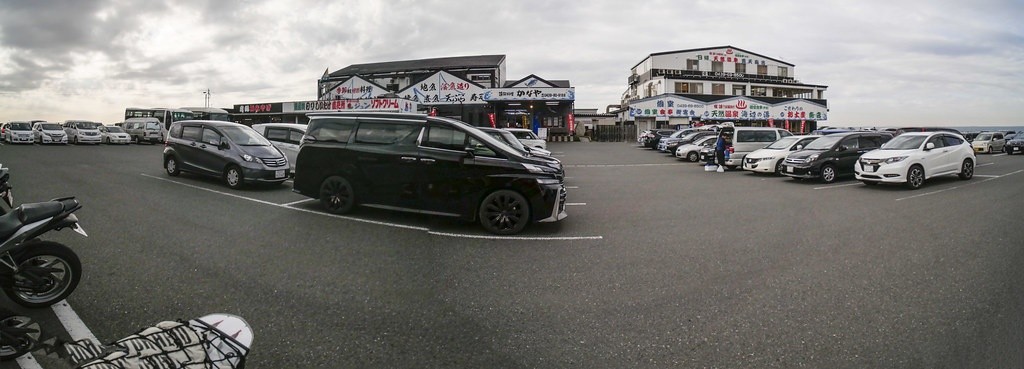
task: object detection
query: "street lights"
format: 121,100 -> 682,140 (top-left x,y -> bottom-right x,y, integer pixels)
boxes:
203,91 -> 207,108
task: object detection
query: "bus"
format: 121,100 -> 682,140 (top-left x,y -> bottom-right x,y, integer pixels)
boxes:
124,107 -> 230,144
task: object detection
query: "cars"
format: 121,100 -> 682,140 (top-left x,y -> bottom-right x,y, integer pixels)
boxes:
0,117 -> 162,145
637,124 -> 1024,190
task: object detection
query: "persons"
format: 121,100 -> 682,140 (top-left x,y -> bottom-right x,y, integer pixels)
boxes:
716,133 -> 729,170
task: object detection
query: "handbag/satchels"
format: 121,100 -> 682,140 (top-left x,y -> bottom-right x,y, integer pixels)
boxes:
717,164 -> 724,172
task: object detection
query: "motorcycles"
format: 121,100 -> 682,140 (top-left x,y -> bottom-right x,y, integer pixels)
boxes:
0,164 -> 88,311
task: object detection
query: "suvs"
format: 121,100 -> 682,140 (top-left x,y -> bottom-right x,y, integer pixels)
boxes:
249,123 -> 336,179
163,120 -> 290,189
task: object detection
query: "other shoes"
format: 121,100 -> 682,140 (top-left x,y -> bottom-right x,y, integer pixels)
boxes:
724,166 -> 728,169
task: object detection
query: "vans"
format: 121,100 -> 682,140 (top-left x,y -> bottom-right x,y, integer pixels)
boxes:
290,113 -> 568,236
